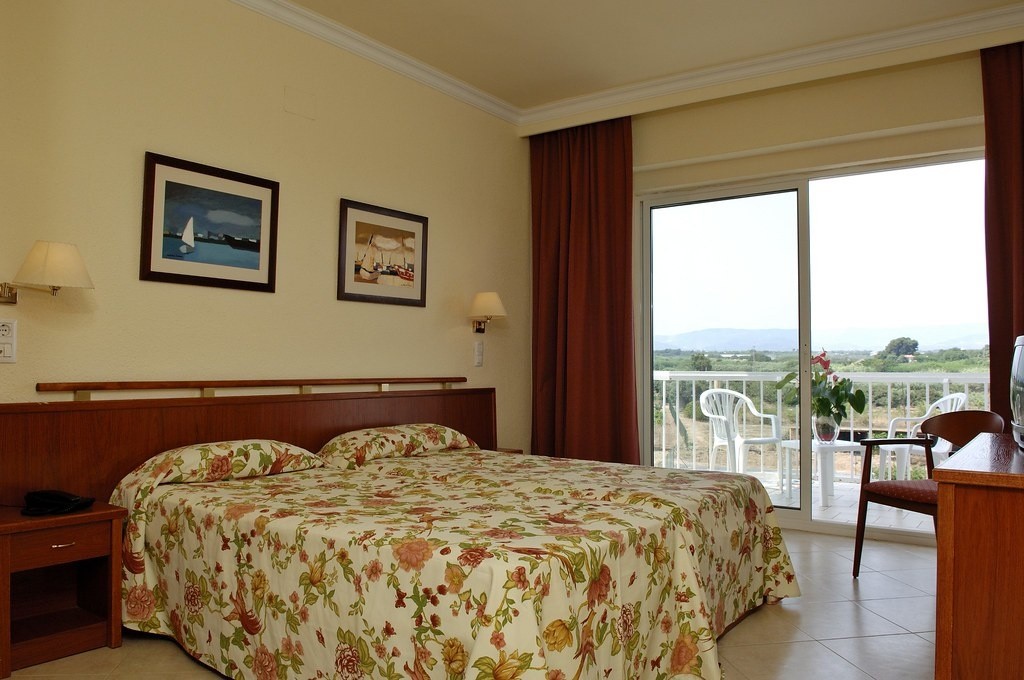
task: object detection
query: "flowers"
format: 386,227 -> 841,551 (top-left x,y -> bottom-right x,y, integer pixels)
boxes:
774,350 -> 867,426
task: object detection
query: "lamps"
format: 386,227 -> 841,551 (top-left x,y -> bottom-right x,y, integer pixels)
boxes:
0,239 -> 95,306
466,291 -> 508,334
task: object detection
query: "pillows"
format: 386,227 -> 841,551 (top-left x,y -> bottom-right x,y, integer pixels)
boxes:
315,423 -> 480,468
130,438 -> 323,484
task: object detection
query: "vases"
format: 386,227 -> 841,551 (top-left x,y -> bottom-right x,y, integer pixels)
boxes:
811,413 -> 840,446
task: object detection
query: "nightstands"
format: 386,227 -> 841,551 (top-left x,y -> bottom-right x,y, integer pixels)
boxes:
1,502 -> 127,679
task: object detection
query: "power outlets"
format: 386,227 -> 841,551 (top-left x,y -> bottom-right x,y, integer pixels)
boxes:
0,318 -> 17,343
474,341 -> 485,356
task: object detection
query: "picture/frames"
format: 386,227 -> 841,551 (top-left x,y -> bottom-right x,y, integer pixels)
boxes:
139,152 -> 280,295
337,197 -> 429,308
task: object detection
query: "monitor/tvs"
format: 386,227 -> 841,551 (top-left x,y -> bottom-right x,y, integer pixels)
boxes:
1009,335 -> 1024,451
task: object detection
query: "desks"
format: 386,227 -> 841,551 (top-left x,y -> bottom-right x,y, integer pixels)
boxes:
778,439 -> 874,508
932,430 -> 1023,680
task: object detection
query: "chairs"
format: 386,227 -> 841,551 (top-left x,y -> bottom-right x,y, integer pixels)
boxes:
877,393 -> 967,484
699,389 -> 785,491
850,409 -> 1005,579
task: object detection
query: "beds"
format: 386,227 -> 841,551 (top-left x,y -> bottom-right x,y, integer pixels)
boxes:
110,422 -> 802,679
